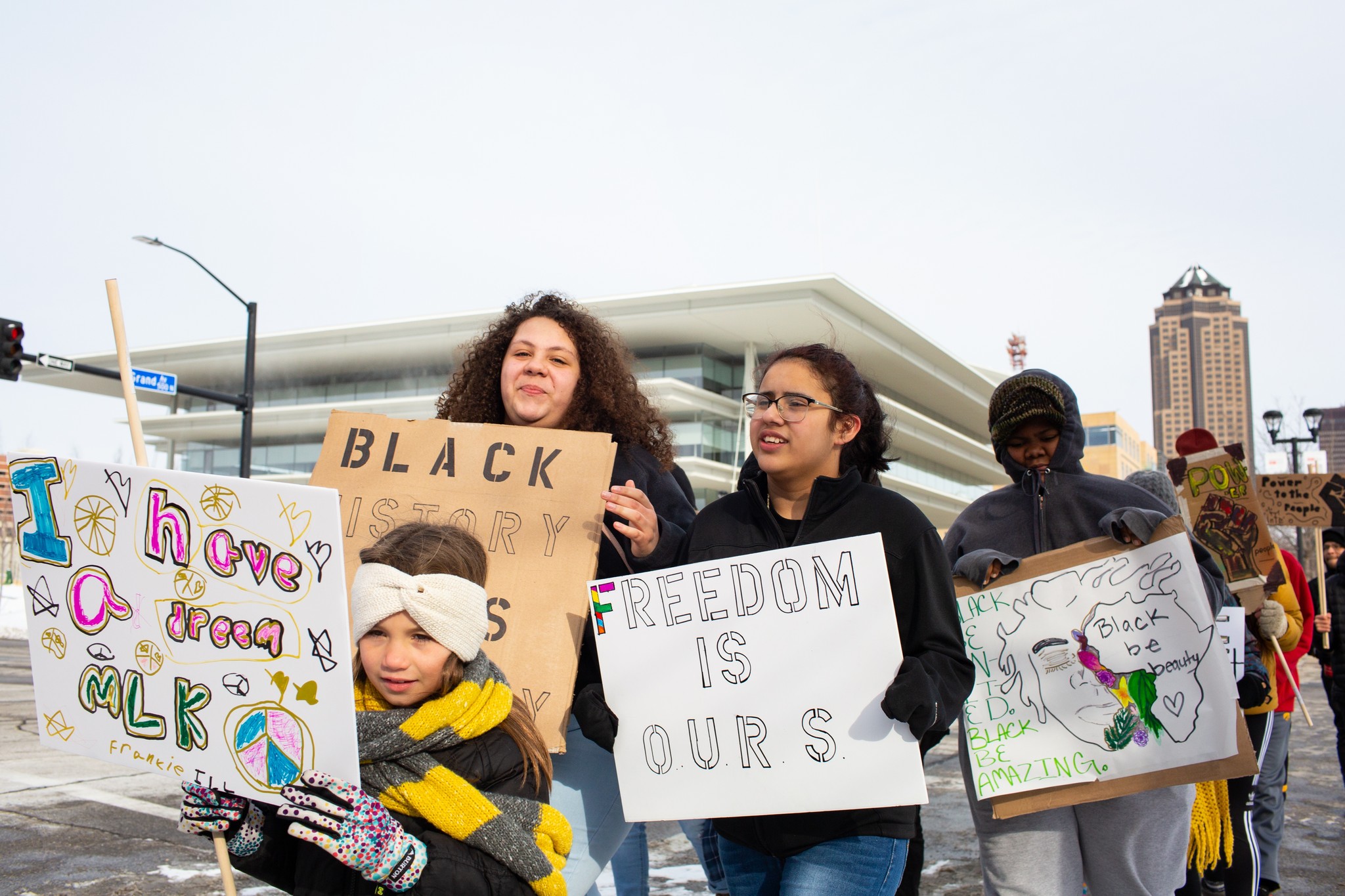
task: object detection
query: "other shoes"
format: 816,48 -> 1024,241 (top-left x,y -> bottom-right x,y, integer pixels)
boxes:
1202,877 -> 1225,892
1257,878 -> 1281,896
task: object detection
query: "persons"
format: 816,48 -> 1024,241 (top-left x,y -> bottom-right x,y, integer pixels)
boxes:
1120,469 -> 1315,896
181,522 -> 572,896
610,820 -> 729,896
673,343 -> 976,896
1307,526 -> 1345,783
434,293 -> 697,896
942,369 -> 1227,896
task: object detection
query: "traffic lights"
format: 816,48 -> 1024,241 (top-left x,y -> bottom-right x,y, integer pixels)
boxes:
0,317 -> 25,382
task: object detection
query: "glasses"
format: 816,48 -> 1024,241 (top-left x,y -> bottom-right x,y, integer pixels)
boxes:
742,393 -> 847,422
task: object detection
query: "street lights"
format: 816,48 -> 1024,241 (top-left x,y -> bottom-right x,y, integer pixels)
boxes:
130,229 -> 259,479
1263,408 -> 1326,572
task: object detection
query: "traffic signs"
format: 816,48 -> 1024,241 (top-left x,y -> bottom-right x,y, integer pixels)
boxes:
36,352 -> 75,374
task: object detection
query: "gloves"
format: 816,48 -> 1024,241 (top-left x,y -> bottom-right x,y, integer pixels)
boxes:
277,771 -> 429,892
1236,672 -> 1268,709
179,780 -> 269,858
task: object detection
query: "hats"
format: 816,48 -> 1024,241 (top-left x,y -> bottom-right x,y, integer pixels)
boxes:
1124,469 -> 1180,515
1322,526 -> 1345,547
991,376 -> 1068,443
